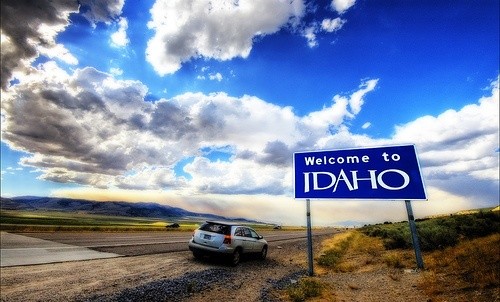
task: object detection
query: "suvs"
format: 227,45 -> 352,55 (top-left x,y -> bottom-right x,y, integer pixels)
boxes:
189,219 -> 269,266
